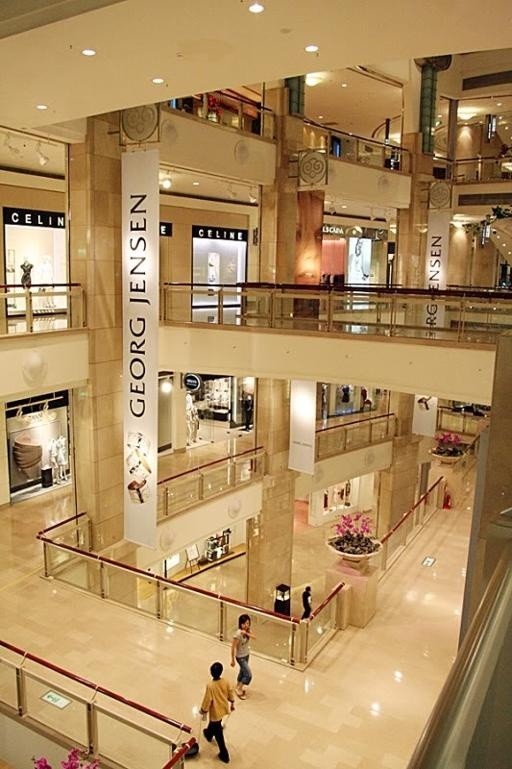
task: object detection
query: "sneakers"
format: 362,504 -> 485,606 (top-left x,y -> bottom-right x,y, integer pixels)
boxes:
235,687 -> 246,700
204,728 -> 212,742
218,753 -> 229,763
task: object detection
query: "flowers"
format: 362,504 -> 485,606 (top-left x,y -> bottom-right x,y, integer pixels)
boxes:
330,509 -> 379,541
432,431 -> 464,456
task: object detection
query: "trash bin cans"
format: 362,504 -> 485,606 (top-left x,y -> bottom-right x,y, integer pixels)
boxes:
42,468 -> 52,488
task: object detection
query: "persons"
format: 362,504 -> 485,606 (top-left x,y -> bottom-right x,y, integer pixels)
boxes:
350,238 -> 364,279
198,661 -> 236,764
239,395 -> 253,430
301,586 -> 313,619
48,439 -> 61,485
57,435 -> 68,481
341,384 -> 349,402
231,613 -> 257,700
20,260 -> 34,290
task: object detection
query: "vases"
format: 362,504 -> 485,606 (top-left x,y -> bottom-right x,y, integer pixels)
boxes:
325,535 -> 383,561
428,448 -> 466,465
